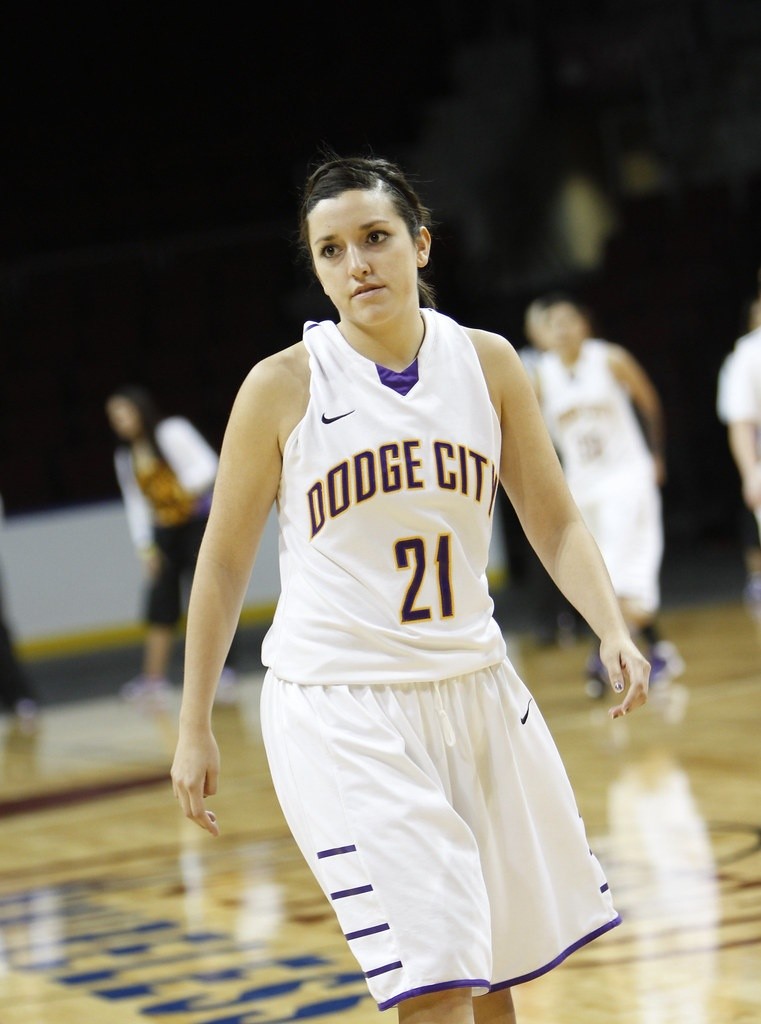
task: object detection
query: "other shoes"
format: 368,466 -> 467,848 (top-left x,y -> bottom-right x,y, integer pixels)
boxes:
587,645 -> 610,701
646,641 -> 681,690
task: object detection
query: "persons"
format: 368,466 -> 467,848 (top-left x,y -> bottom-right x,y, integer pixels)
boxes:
169,159 -> 652,1024
107,385 -> 240,704
718,323 -> 760,518
516,296 -> 684,700
